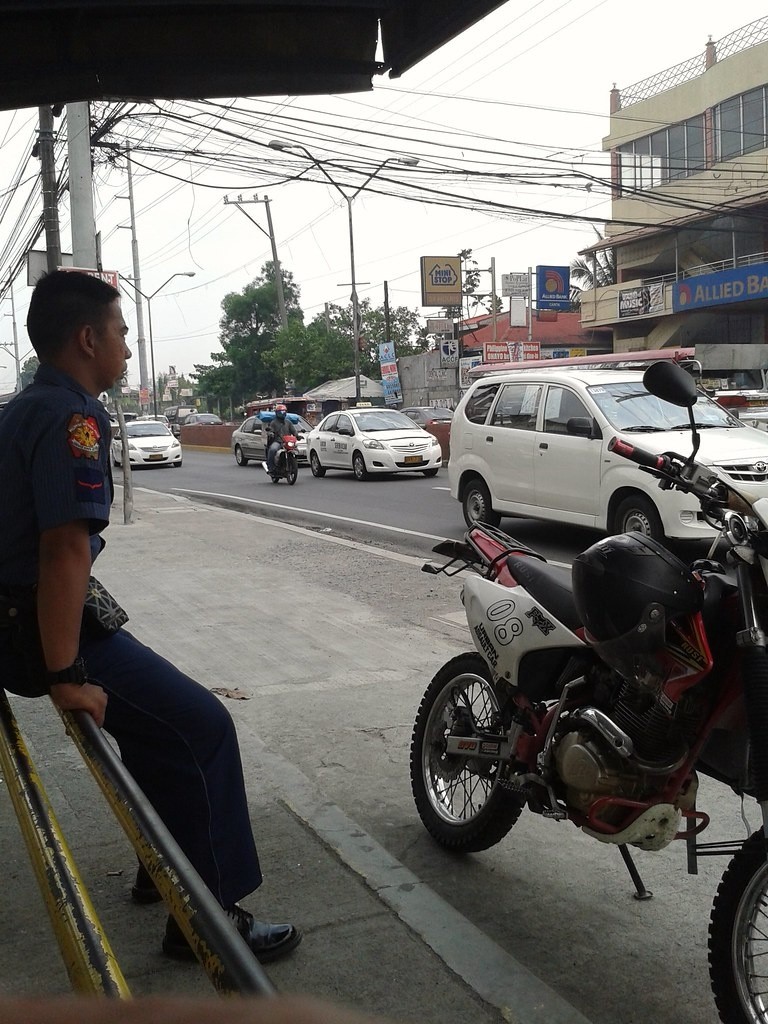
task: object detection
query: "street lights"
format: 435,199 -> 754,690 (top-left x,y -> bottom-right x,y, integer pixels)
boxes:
268,138 -> 421,409
112,271 -> 195,419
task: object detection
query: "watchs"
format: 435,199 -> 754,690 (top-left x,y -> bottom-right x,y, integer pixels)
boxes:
45,657 -> 87,686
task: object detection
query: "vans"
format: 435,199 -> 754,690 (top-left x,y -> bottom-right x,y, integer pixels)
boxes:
446,369 -> 768,548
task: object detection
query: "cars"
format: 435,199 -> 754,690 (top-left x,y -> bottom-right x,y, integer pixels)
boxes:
112,420 -> 183,467
232,412 -> 318,466
108,405 -> 232,429
306,401 -> 444,481
398,406 -> 456,431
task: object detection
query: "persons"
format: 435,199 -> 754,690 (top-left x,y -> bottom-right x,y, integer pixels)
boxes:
0,269 -> 301,962
267,405 -> 305,475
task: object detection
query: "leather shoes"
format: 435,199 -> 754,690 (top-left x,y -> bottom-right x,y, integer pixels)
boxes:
163,903 -> 302,962
131,864 -> 165,904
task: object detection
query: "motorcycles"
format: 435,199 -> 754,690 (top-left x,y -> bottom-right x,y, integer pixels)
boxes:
409,355 -> 768,1024
260,426 -> 306,486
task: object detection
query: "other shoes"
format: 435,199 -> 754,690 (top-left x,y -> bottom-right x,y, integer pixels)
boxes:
268,469 -> 275,473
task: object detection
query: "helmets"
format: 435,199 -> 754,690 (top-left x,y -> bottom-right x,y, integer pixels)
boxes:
276,405 -> 287,418
573,531 -> 704,691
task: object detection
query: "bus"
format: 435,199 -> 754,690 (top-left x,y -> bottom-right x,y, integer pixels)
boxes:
245,397 -> 317,425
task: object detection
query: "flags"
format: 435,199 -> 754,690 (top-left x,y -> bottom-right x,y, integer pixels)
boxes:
379,342 -> 404,406
